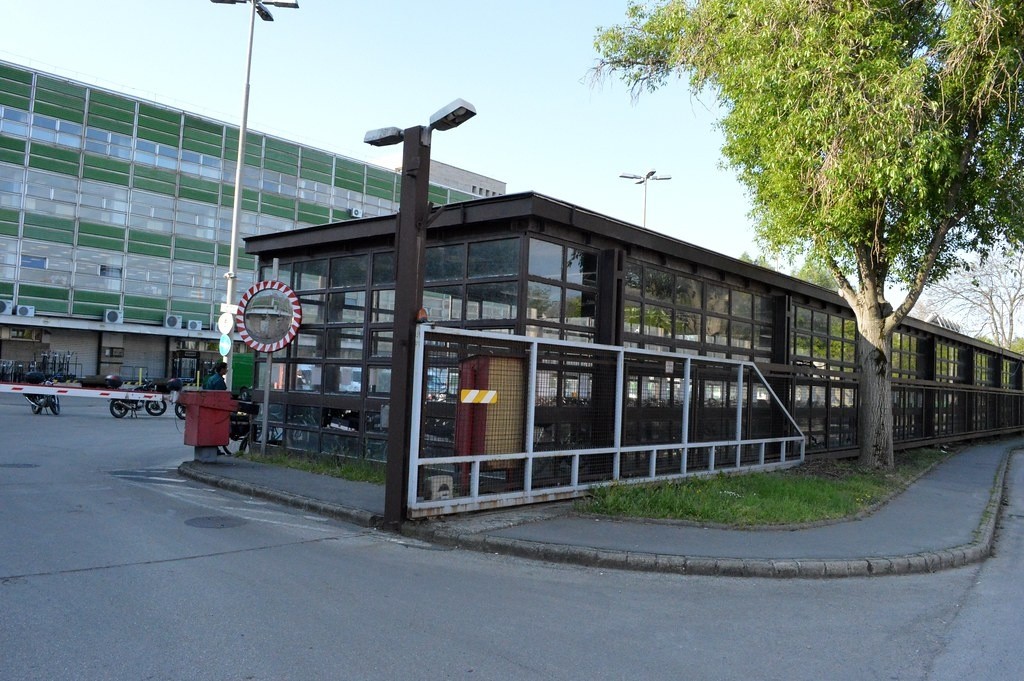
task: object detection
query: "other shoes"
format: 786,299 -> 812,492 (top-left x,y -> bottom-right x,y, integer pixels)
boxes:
217,450 -> 226,455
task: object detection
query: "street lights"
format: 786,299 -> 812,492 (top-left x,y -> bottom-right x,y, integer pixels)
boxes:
210,0 -> 299,387
363,98 -> 477,531
619,168 -> 672,228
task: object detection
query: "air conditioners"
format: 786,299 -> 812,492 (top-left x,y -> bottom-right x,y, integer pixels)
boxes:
163,315 -> 183,329
213,322 -> 220,332
103,308 -> 123,324
187,320 -> 203,330
15,305 -> 34,316
0,299 -> 12,315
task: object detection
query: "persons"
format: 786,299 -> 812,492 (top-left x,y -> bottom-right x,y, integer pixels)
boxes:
203,361 -> 233,456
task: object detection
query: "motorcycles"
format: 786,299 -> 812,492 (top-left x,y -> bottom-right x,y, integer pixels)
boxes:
104,370 -> 167,419
23,371 -> 64,415
